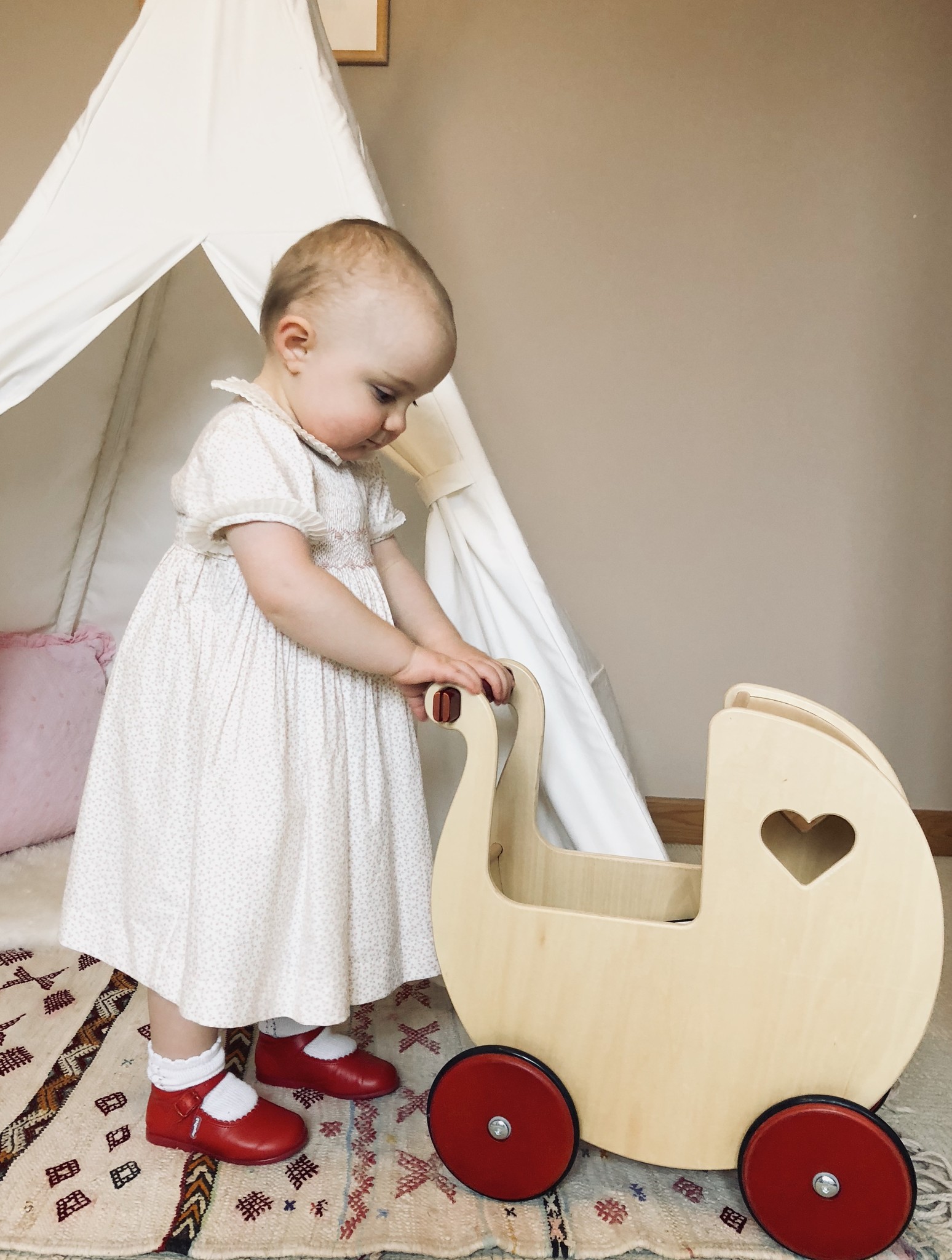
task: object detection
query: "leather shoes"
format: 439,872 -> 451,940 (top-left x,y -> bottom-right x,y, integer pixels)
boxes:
145,1070 -> 310,1167
255,1027 -> 400,1099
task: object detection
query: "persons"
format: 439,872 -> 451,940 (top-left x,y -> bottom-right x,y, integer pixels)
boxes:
50,214 -> 516,1162
